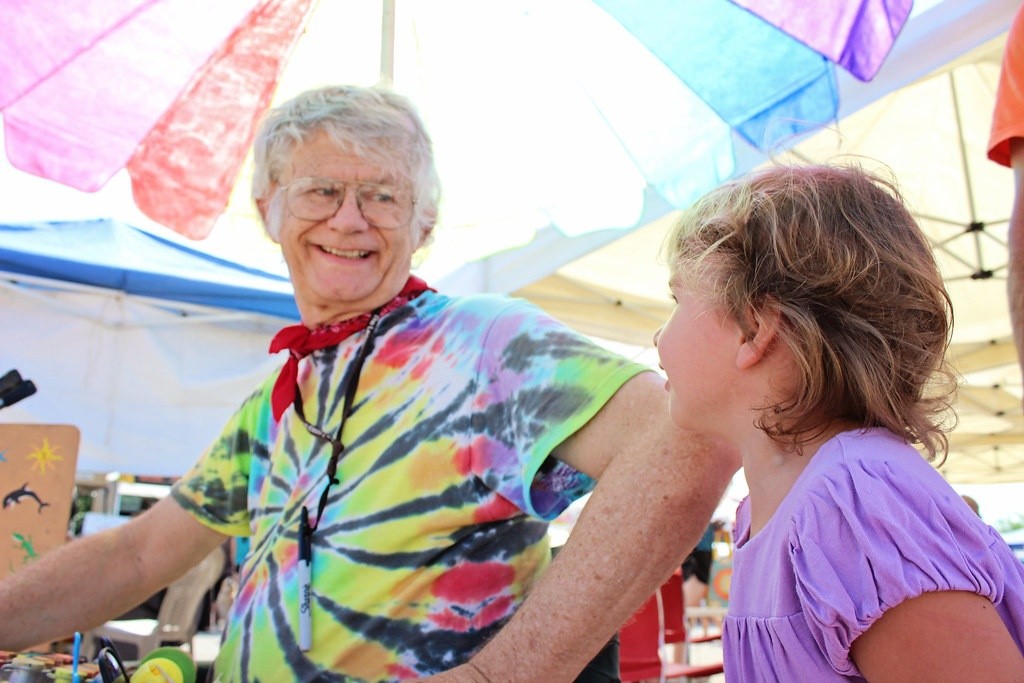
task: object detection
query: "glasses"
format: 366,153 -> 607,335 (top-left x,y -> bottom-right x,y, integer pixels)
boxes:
279,177 -> 419,230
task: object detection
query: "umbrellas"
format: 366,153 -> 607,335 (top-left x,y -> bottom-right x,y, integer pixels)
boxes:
1,0 -> 911,286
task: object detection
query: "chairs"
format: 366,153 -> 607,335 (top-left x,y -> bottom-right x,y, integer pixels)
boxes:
618,563 -> 725,683
82,546 -> 226,672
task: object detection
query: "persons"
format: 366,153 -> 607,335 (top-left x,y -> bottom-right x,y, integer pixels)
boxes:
654,164 -> 1023,683
0,83 -> 742,681
986,10 -> 1023,390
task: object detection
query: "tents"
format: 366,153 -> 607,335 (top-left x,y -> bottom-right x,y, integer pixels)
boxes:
1,215 -> 305,483
